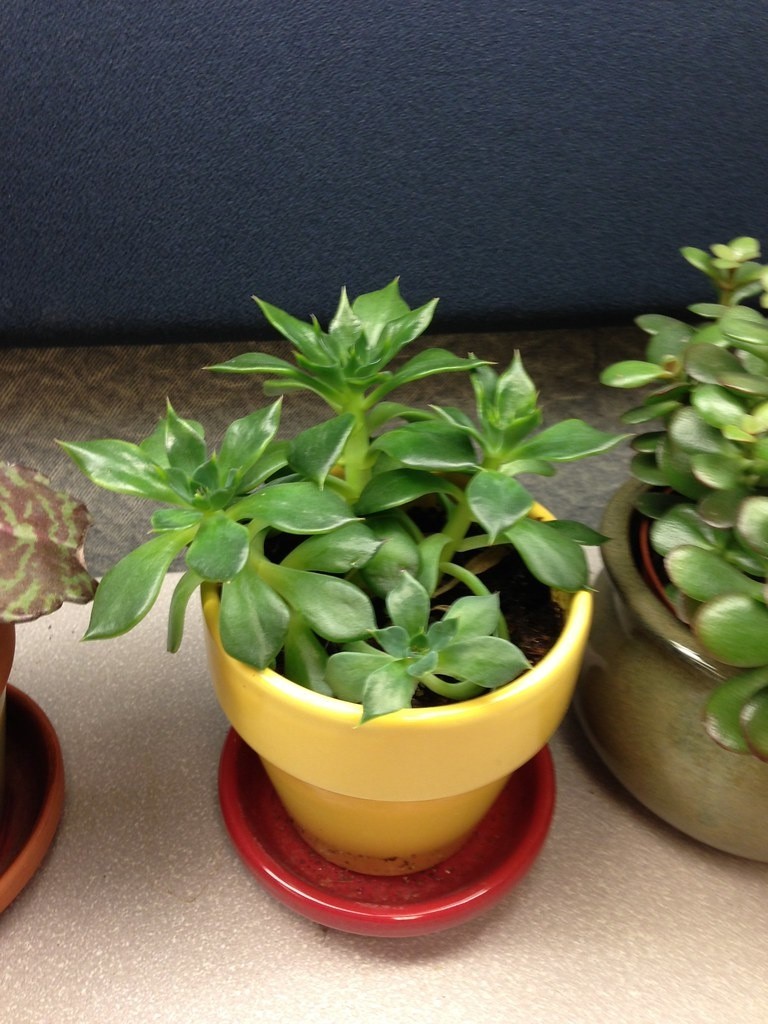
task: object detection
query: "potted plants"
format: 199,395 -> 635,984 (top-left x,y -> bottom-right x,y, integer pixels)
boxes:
572,233 -> 768,864
46,274 -> 637,882
0,461 -> 102,916
